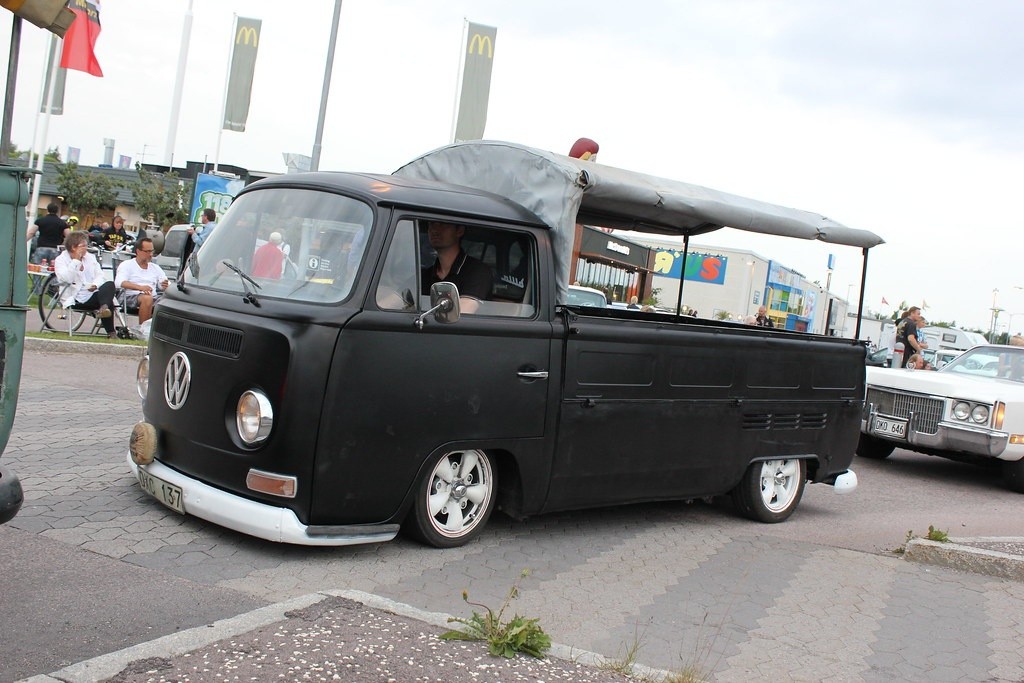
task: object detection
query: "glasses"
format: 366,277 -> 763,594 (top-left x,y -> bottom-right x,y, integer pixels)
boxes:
141,249 -> 155,254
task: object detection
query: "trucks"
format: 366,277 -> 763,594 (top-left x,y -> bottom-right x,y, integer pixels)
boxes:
128,140 -> 884,548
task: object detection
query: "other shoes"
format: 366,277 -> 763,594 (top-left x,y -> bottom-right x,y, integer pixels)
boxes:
96,309 -> 121,340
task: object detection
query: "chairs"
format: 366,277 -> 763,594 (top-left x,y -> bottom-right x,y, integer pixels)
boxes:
40,282 -> 110,337
481,272 -> 528,316
116,287 -> 140,335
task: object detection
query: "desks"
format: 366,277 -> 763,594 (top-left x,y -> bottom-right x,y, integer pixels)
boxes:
28,270 -> 57,302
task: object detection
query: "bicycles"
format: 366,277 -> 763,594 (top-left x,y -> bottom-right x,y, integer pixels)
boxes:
39,239 -> 135,333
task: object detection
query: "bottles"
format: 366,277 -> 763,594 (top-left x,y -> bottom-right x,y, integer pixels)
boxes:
40,258 -> 47,273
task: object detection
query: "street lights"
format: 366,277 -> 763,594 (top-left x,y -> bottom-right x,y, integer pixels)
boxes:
989,307 -> 1004,343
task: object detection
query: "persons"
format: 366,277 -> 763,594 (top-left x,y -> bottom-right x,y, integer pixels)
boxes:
915,316 -> 928,358
66,215 -> 79,233
376,221 -> 483,313
923,361 -> 931,369
27,203 -> 70,294
274,228 -> 290,279
887,318 -> 903,368
102,222 -> 109,237
639,305 -> 656,313
895,306 -> 928,368
692,310 -> 697,317
250,231 -> 282,280
688,309 -> 693,315
627,296 -> 640,309
602,288 -> 612,304
755,305 -> 774,327
55,232 -> 120,340
744,315 -> 758,326
115,238 -> 171,324
901,312 -> 908,319
88,220 -> 103,233
907,353 -> 923,369
186,209 -> 216,254
104,215 -> 126,279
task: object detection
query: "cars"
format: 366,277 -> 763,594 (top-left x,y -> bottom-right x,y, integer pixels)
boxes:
858,343 -> 1022,488
156,222 -> 192,284
